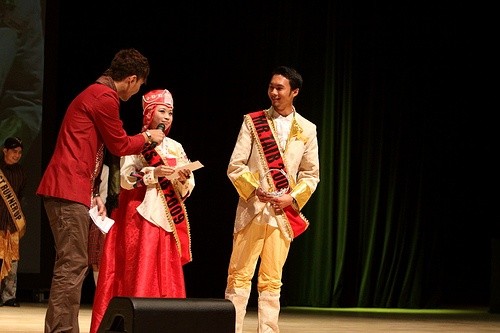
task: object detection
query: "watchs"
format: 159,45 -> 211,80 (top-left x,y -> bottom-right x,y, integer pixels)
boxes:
145,130 -> 151,143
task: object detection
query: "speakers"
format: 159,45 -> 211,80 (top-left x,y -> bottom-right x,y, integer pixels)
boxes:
97,295 -> 236,333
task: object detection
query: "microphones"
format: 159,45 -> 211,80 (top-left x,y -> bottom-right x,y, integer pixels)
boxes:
148,123 -> 165,150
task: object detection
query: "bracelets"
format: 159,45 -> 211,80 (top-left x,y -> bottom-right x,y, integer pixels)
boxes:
93,192 -> 100,196
292,196 -> 296,204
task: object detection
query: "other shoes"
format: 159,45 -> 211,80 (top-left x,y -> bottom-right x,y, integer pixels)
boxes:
4,298 -> 19,307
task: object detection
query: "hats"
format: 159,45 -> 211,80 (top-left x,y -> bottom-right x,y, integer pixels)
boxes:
3,137 -> 23,150
142,89 -> 174,116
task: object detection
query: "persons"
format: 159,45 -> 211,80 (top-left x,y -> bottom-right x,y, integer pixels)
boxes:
82,162 -> 110,289
35,47 -> 152,333
90,87 -> 197,333
0,136 -> 30,307
226,64 -> 320,332
